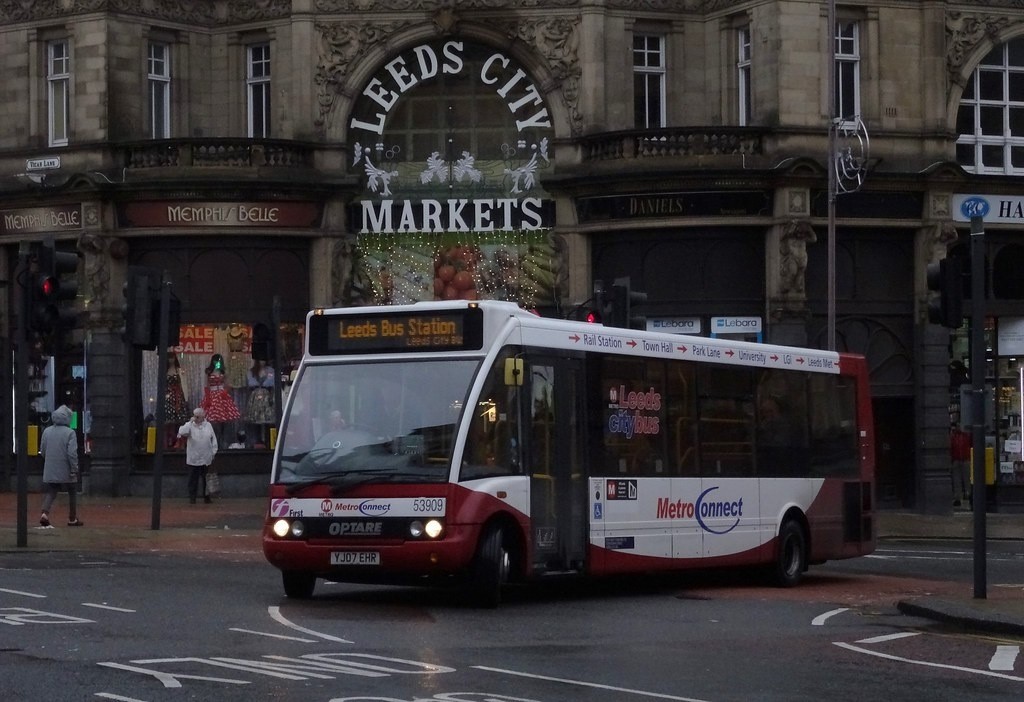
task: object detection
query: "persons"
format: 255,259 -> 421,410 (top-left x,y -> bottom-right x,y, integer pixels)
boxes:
950,424 -> 972,506
167,353 -> 188,423
178,407 -> 218,504
490,420 -> 512,468
464,417 -> 490,466
246,353 -> 275,443
927,223 -> 958,274
227,326 -> 250,405
40,405 -> 85,526
780,218 -> 817,294
201,354 -> 239,447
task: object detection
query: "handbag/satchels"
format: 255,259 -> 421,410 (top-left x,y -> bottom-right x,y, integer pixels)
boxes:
205,473 -> 221,494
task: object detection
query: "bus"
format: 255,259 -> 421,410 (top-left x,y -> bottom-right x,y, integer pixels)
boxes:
262,299 -> 876,610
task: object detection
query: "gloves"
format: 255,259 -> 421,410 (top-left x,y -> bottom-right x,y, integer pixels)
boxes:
70,472 -> 77,477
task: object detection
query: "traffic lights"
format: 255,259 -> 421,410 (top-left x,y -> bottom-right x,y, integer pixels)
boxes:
614,278 -> 648,331
25,259 -> 61,333
573,305 -> 600,323
118,268 -> 163,350
925,257 -> 958,329
268,425 -> 277,451
146,426 -> 156,454
26,425 -> 40,457
48,248 -> 84,331
250,323 -> 274,361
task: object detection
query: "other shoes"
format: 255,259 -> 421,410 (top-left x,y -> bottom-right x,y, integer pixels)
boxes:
68,518 -> 83,526
190,497 -> 195,503
204,497 -> 213,503
40,513 -> 50,526
